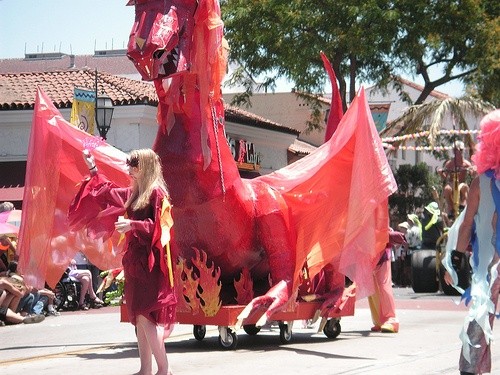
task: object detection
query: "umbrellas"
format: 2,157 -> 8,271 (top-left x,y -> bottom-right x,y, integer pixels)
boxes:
379,97 -> 496,189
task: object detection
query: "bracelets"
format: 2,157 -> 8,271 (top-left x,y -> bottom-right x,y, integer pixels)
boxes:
89,165 -> 96,170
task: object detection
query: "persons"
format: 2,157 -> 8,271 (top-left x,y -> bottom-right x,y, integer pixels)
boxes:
67,148 -> 178,375
0,110 -> 500,375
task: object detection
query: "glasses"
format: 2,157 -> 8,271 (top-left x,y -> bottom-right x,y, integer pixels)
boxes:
126,157 -> 141,167
453,148 -> 464,151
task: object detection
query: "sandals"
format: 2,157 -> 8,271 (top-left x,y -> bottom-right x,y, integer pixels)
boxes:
79,303 -> 89,311
89,297 -> 105,305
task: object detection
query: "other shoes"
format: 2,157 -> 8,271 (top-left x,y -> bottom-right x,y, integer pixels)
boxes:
22,315 -> 45,324
48,310 -> 61,316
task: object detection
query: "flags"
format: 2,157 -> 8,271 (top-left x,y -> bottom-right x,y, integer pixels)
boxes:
70,87 -> 96,136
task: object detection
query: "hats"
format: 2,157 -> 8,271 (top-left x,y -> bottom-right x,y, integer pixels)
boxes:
425,201 -> 440,216
408,213 -> 419,224
398,222 -> 409,229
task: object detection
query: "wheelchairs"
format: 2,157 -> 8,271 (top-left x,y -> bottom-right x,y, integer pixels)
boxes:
45,270 -> 103,312
